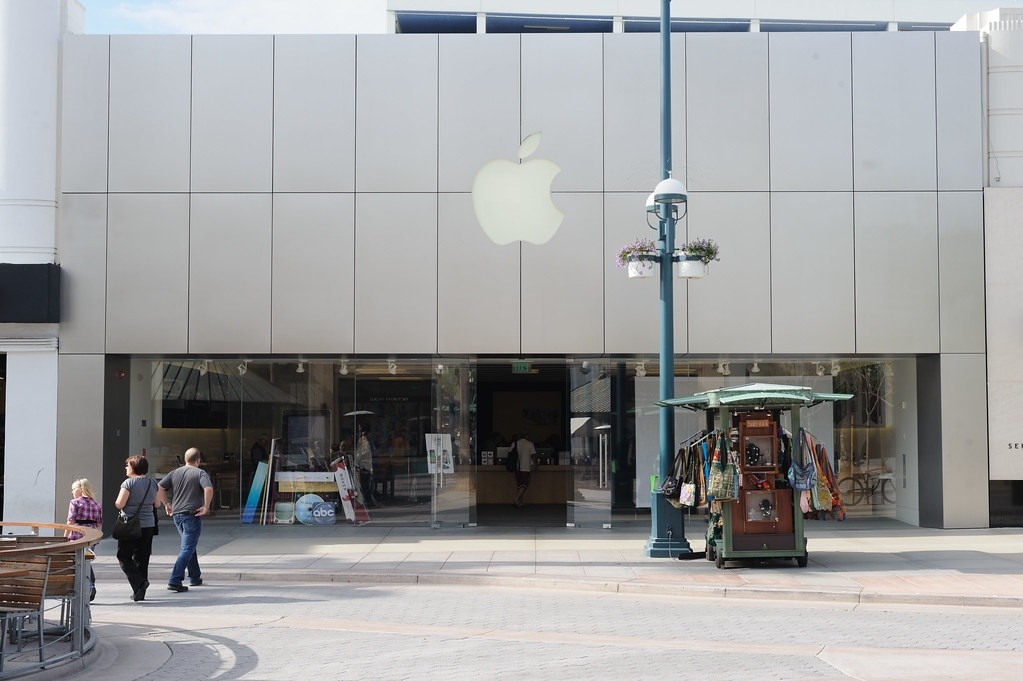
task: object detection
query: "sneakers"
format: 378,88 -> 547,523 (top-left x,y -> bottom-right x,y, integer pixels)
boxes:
190,578 -> 202,586
167,584 -> 188,592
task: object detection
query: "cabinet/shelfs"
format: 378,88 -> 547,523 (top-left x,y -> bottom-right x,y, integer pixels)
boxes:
704,405 -> 806,559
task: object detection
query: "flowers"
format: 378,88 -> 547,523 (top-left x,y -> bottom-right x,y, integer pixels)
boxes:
616,236 -> 659,275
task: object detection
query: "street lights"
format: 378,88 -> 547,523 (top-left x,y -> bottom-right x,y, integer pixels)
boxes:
616,0 -> 722,559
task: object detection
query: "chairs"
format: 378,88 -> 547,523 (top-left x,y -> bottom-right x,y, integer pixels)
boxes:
160,464 -> 174,474
0,555 -> 52,673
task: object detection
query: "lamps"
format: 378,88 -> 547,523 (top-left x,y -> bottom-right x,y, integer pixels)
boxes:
811,361 -> 826,377
435,364 -> 444,375
830,359 -> 841,377
751,363 -> 760,372
386,360 -> 398,375
236,360 -> 254,375
198,360 -> 215,376
722,363 -> 731,376
717,363 -> 725,373
339,360 -> 350,375
634,362 -> 647,377
295,360 -> 310,373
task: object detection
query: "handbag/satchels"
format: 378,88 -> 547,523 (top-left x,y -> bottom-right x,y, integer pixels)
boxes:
705,502 -> 723,546
783,427 -> 847,522
506,440 -> 519,473
112,514 -> 141,540
660,432 -> 741,510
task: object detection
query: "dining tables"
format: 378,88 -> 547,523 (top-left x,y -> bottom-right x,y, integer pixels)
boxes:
198,461 -> 231,511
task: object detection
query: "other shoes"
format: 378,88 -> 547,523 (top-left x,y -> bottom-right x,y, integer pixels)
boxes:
89,587 -> 96,601
513,498 -> 523,506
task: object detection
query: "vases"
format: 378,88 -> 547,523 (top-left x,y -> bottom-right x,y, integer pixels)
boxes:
682,237 -> 720,276
678,259 -> 705,278
627,260 -> 656,279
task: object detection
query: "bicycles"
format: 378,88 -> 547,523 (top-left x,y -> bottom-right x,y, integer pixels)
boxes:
837,458 -> 898,506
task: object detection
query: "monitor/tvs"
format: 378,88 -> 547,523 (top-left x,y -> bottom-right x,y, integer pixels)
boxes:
283,409 -> 331,470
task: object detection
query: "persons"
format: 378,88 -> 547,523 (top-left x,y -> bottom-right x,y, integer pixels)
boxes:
64,477 -> 103,602
158,447 -> 213,592
115,455 -> 161,601
250,429 -> 416,507
509,430 -> 537,507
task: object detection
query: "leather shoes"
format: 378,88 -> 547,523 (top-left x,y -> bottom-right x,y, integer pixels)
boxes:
130,593 -> 144,599
133,581 -> 149,602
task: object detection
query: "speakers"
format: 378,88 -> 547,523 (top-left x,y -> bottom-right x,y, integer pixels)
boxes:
0,263 -> 61,324
